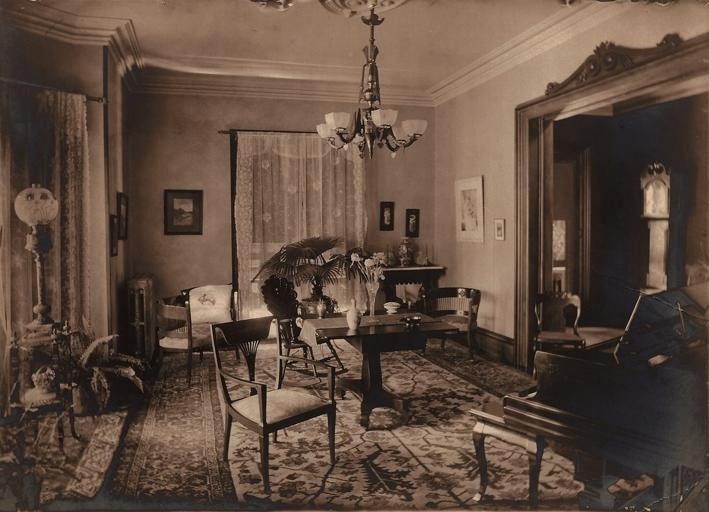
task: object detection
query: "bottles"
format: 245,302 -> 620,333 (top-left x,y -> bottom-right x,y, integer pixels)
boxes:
346,297 -> 361,331
397,237 -> 414,267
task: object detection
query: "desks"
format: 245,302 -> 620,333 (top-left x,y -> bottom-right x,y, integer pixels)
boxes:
295,312 -> 459,426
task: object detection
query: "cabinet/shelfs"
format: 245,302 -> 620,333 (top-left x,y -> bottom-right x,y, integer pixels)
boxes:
11,335 -> 78,460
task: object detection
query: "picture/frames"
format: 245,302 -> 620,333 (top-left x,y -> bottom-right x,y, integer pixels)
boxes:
455,175 -> 485,243
110,212 -> 119,255
405,209 -> 420,237
495,219 -> 505,240
379,200 -> 394,231
163,188 -> 204,235
115,192 -> 128,240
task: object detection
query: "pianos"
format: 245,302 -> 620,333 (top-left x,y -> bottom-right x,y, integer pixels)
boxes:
503,281 -> 708,512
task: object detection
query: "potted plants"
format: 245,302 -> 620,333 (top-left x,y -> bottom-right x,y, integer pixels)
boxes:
38,313 -> 156,415
250,234 -> 368,314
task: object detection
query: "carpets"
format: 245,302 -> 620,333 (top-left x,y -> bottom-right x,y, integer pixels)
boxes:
115,337 -> 700,511
28,406 -> 131,505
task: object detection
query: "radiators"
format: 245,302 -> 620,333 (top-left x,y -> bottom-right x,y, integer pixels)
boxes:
127,272 -> 155,357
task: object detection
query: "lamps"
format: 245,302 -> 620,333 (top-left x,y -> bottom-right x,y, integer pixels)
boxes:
314,0 -> 429,160
13,179 -> 60,348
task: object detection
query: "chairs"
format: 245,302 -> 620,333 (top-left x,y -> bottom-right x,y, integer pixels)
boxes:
527,290 -> 586,379
208,316 -> 336,494
260,273 -> 344,376
185,282 -> 240,361
421,285 -> 480,357
150,297 -> 226,384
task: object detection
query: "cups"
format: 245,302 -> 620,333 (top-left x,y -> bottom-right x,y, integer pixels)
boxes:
399,313 -> 423,326
315,299 -> 327,318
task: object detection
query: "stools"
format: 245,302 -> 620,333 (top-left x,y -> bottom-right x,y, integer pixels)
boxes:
467,401 -> 549,511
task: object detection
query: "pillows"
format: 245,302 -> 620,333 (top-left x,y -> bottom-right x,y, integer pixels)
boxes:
189,284 -> 234,323
